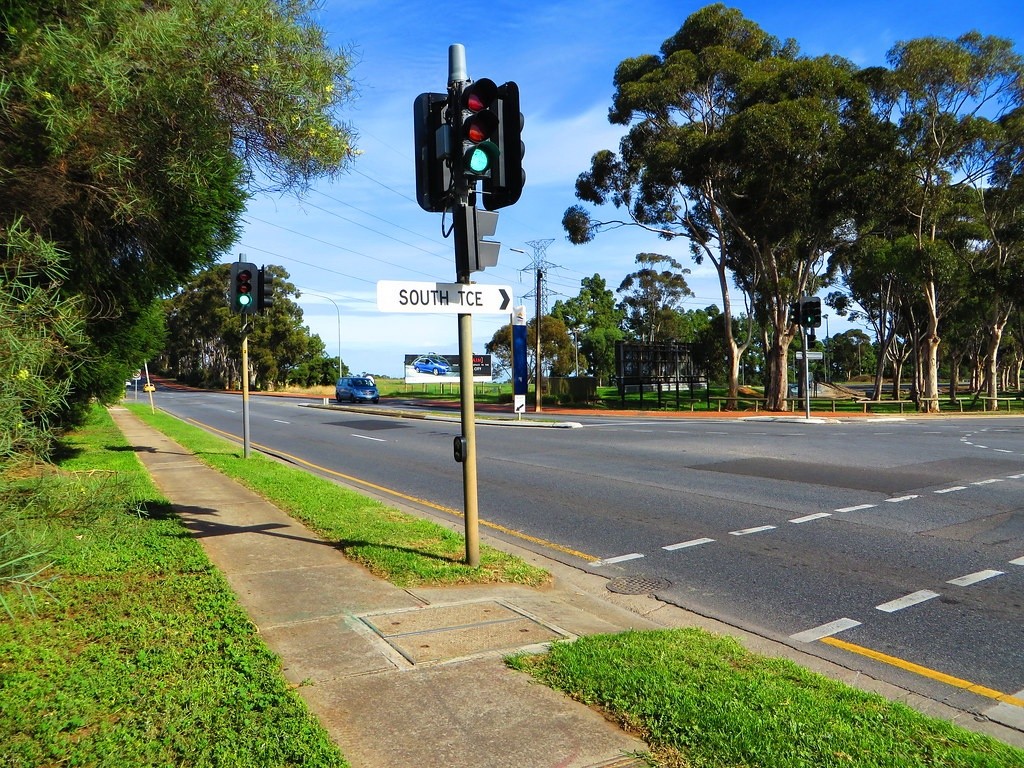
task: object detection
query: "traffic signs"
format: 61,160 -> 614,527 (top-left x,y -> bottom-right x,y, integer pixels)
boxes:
376,280 -> 514,314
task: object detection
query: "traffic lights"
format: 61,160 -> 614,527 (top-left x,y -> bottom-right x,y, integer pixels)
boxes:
461,77 -> 502,178
264,273 -> 274,307
237,270 -> 253,307
789,301 -> 799,324
805,300 -> 815,326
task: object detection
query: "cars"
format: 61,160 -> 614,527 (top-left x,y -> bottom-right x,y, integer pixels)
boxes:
413,358 -> 450,376
335,376 -> 379,404
143,384 -> 155,392
126,379 -> 131,387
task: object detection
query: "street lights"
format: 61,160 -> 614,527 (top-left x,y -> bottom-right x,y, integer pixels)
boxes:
295,292 -> 342,378
